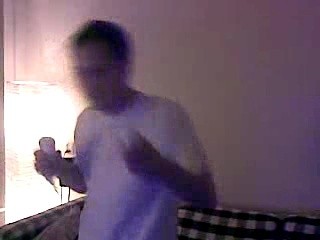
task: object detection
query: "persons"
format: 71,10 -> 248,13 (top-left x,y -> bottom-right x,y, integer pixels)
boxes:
33,19 -> 217,240
